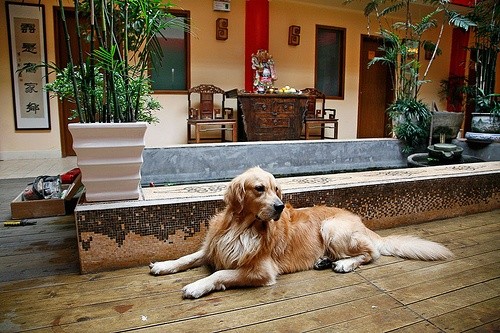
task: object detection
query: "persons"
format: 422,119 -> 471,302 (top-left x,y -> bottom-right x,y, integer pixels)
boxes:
251,49 -> 277,92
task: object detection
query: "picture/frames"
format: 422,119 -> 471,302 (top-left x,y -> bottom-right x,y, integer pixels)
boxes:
400,38 -> 420,103
5,1 -> 52,131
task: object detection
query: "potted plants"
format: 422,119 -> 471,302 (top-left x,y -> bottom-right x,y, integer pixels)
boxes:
21,0 -> 200,202
464,0 -> 500,134
344,0 -> 477,138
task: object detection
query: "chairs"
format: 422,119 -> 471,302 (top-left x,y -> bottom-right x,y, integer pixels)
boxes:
187,84 -> 237,143
301,88 -> 339,139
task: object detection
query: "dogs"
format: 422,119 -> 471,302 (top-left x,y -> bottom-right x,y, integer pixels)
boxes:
149,164 -> 459,300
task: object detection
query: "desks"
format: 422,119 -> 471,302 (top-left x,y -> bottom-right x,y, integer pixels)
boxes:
224,89 -> 315,142
